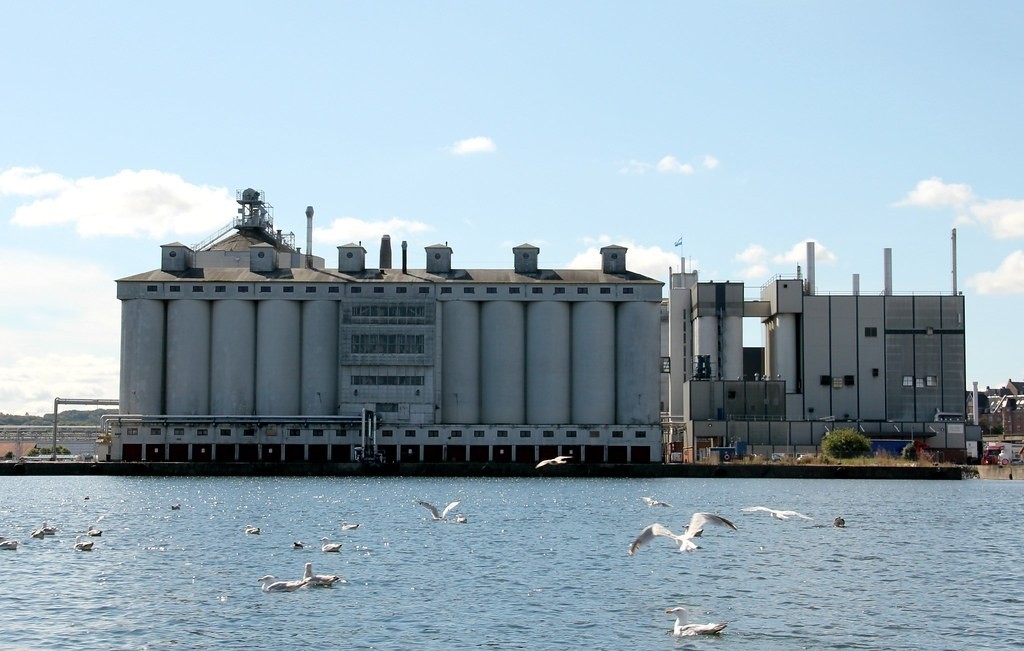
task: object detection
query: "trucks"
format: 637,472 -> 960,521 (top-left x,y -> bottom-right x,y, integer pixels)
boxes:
981,441 -> 1013,465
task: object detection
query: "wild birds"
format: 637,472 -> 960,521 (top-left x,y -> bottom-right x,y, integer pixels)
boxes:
629,512 -> 738,555
245,525 -> 261,535
742,506 -> 812,521
257,575 -> 308,594
30,521 -> 56,539
419,501 -> 467,523
303,563 -> 340,588
666,607 -> 729,637
75,525 -> 102,550
340,521 -> 359,531
535,456 -> 572,469
642,497 -> 672,507
85,496 -> 90,500
0,536 -> 19,551
294,541 -> 303,548
832,516 -> 845,527
172,504 -> 181,510
319,537 -> 342,552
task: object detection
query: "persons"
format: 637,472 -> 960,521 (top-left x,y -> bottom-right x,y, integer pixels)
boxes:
998,450 -> 1007,465
